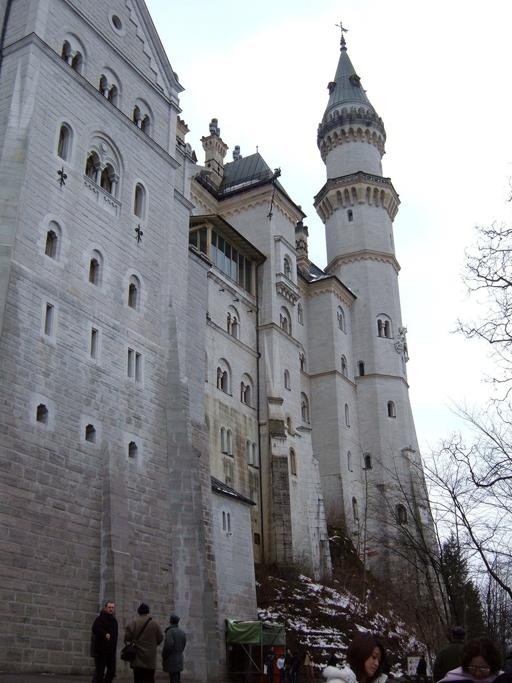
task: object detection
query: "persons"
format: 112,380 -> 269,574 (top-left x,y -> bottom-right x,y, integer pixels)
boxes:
431,625 -> 466,682
321,632 -> 389,683
89,600 -> 119,682
263,643 -> 316,683
163,614 -> 187,683
415,654 -> 429,682
434,636 -> 505,682
124,601 -> 165,683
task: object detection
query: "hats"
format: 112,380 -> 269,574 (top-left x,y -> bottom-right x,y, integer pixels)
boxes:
137,602 -> 150,615
169,614 -> 180,624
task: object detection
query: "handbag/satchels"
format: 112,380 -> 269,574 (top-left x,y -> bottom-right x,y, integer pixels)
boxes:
120,642 -> 136,662
